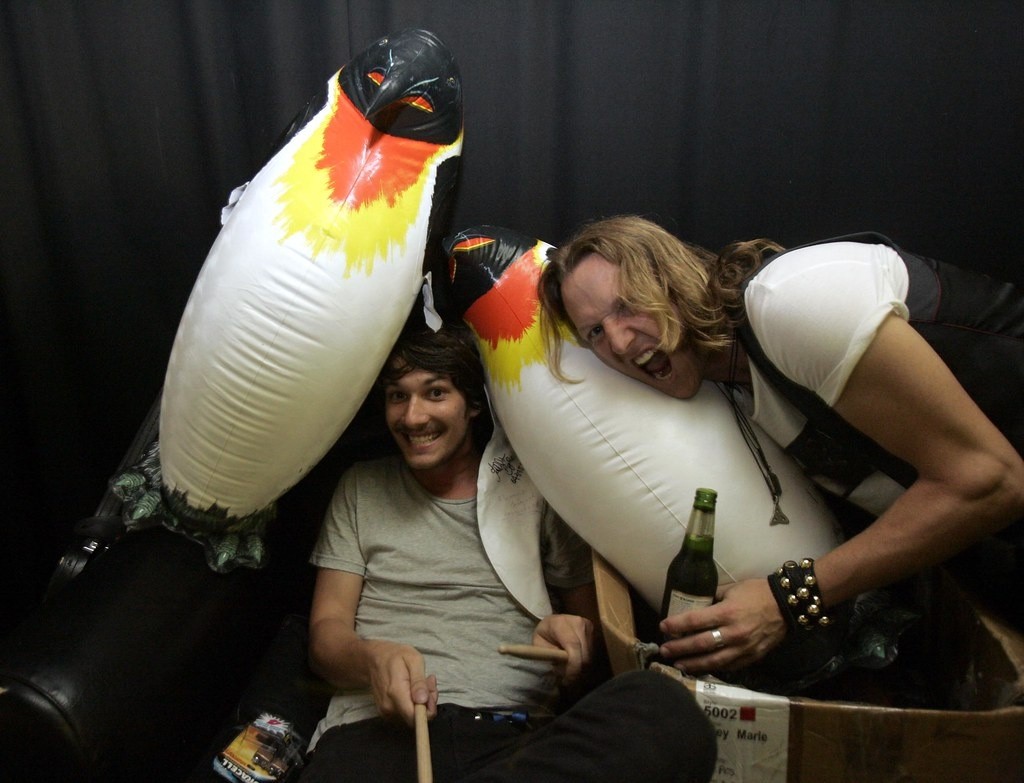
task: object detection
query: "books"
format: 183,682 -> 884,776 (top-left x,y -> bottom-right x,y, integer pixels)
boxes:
214,708 -> 309,783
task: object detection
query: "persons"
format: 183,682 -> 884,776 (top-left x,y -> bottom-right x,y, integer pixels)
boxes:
294,322 -> 717,782
540,215 -> 1024,675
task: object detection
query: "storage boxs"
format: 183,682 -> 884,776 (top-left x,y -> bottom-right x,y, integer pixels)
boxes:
589,548 -> 1024,783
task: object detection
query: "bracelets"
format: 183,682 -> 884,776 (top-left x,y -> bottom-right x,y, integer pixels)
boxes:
768,558 -> 840,643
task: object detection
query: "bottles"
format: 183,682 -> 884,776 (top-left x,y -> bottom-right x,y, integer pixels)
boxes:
656,487 -> 719,664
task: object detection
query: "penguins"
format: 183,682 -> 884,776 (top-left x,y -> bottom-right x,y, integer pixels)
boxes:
107,26 -> 465,570
443,223 -> 845,620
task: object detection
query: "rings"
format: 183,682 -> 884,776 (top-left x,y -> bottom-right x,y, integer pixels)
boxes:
712,629 -> 723,648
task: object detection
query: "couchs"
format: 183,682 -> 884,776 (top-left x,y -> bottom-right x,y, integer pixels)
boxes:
0,388 -> 659,783
